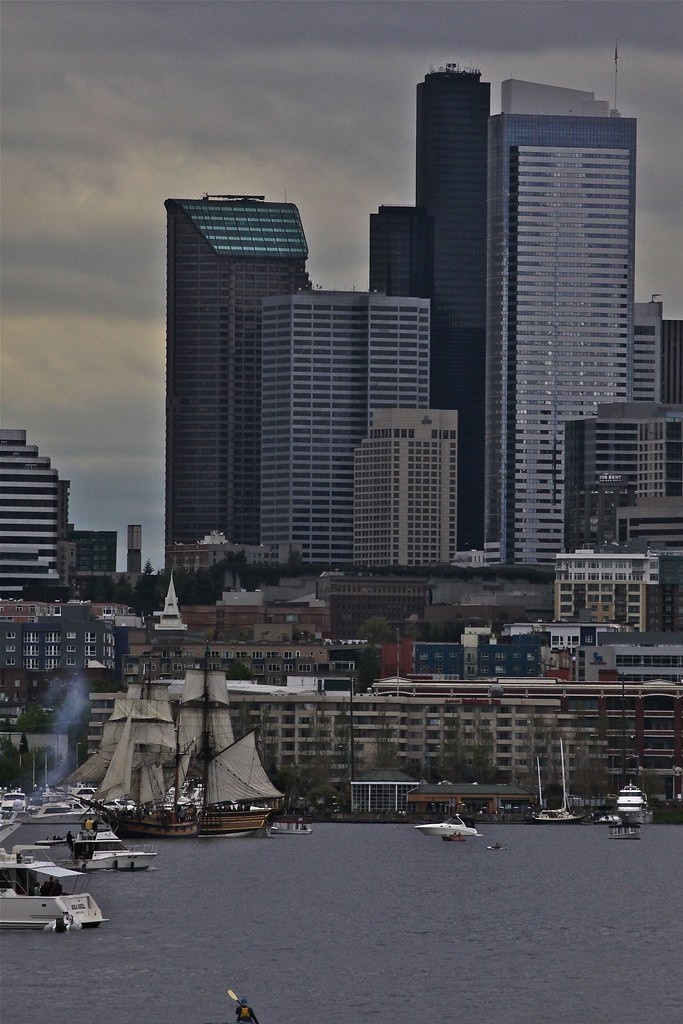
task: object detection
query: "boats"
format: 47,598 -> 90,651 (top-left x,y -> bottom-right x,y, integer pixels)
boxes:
269,816 -> 312,835
593,814 -> 625,827
0,642 -> 288,932
616,778 -> 655,824
413,812 -> 482,838
525,737 -> 586,823
608,825 -> 642,840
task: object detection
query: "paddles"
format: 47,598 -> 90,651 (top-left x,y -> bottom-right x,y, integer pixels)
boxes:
227,989 -> 243,1006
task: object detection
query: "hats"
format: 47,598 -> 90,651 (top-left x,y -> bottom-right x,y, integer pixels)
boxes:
240,999 -> 247,1005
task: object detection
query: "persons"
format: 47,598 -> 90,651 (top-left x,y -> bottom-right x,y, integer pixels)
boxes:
66,817 -> 99,860
102,804 -> 249,825
453,831 -> 461,838
495,842 -> 501,848
303,825 -> 306,830
45,836 -> 62,840
34,876 -> 63,897
